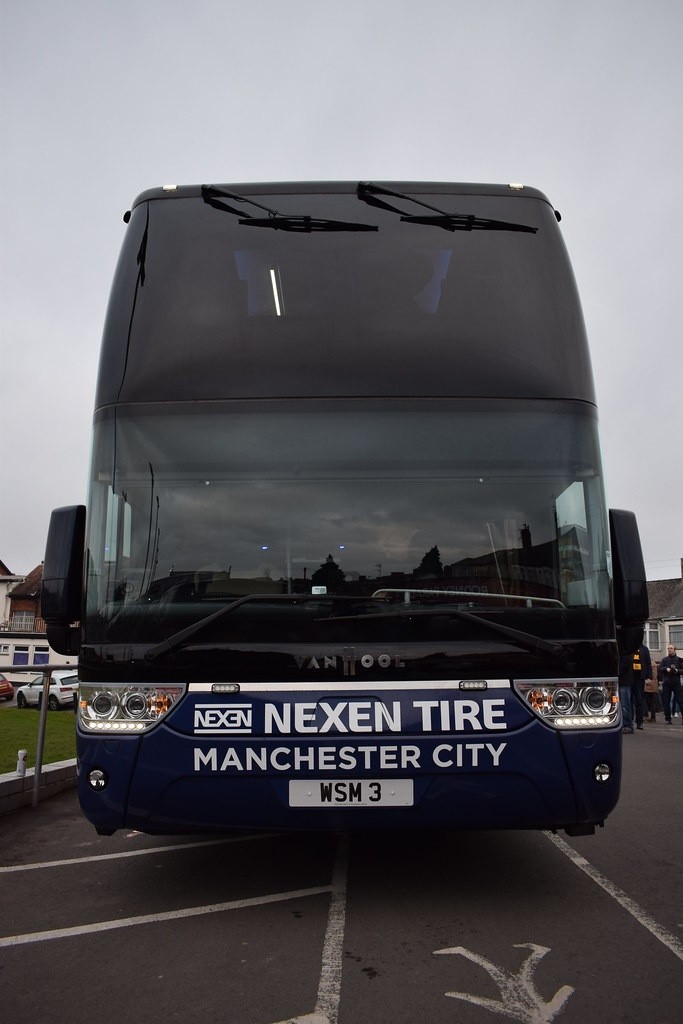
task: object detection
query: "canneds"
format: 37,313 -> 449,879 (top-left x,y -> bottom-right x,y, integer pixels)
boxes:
17,749 -> 27,777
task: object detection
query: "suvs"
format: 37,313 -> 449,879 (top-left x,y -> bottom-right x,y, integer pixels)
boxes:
15,670 -> 80,711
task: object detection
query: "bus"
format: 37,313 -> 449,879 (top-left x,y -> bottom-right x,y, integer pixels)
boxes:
39,180 -> 649,857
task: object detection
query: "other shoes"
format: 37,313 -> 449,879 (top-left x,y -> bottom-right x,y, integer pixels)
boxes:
623,727 -> 632,733
674,713 -> 678,718
651,718 -> 655,722
636,724 -> 642,729
643,718 -> 648,722
665,720 -> 671,724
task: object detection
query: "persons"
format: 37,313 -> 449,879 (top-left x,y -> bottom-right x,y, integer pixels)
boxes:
618,642 -> 683,734
373,520 -> 448,599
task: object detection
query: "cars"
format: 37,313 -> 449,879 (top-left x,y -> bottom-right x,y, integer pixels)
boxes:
0,673 -> 15,701
